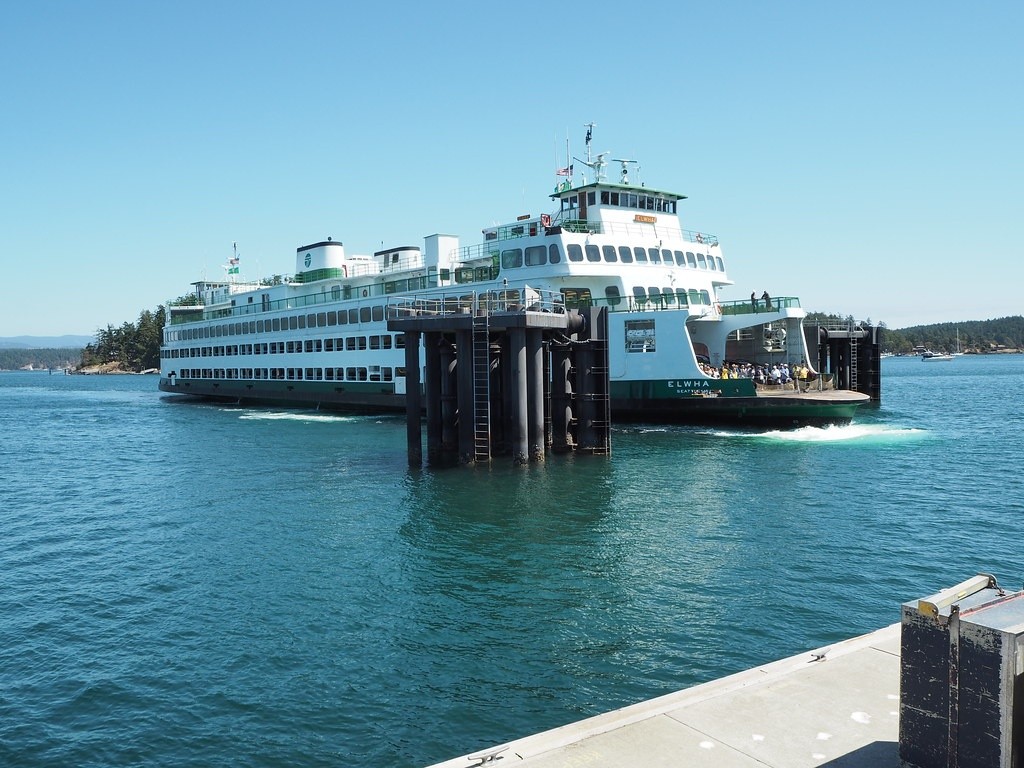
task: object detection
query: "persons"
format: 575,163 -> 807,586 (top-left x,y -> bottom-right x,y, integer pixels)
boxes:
761,291 -> 773,312
698,360 -> 810,392
751,291 -> 759,313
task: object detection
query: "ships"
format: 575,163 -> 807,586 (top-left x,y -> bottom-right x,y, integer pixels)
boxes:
157,120 -> 874,428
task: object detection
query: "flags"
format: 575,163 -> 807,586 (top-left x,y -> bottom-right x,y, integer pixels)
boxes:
556,164 -> 573,176
540,213 -> 550,227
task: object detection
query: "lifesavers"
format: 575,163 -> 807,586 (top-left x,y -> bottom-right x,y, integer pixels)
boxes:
362,289 -> 368,298
696,234 -> 703,243
540,214 -> 550,227
